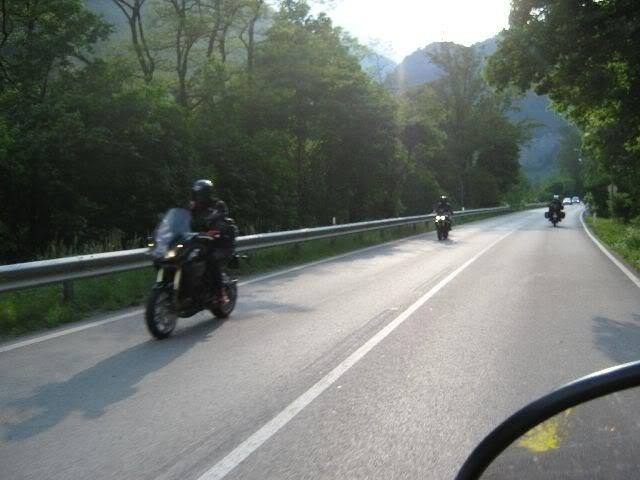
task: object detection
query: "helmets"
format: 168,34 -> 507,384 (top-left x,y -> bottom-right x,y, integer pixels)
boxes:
192,180 -> 215,200
440,196 -> 448,204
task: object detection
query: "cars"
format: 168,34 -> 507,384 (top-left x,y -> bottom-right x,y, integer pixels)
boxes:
563,196 -> 579,205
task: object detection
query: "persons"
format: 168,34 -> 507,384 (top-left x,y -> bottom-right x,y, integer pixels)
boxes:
546,193 -> 565,222
433,195 -> 454,230
159,178 -> 238,309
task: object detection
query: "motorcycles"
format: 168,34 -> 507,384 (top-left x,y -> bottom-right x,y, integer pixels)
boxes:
146,207 -> 247,340
549,205 -> 562,226
433,205 -> 451,240
